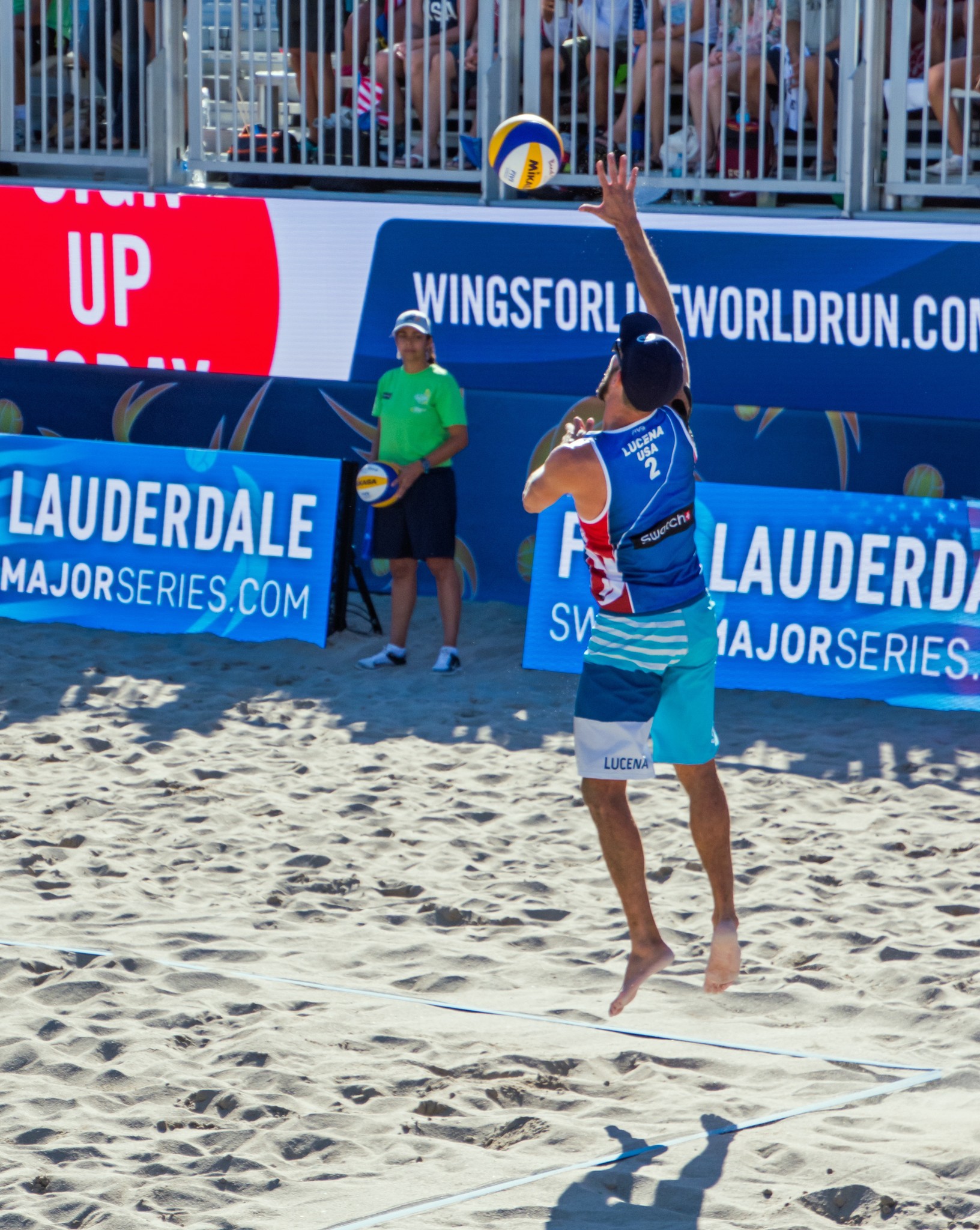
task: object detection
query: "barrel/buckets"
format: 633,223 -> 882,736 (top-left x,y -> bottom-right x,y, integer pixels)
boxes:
715,114 -> 776,207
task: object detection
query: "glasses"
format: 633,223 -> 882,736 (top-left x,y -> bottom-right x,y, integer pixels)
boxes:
610,338 -> 621,366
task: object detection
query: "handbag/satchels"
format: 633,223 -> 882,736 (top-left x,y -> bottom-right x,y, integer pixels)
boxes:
308,126 -> 387,193
226,124 -> 300,189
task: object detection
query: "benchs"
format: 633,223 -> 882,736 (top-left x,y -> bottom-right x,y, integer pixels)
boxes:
254,72 -> 980,161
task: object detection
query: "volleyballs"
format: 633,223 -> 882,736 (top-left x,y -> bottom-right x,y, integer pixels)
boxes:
487,113 -> 564,190
356,460 -> 402,508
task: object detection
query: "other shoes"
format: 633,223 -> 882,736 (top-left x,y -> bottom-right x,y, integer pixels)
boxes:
804,158 -> 836,175
928,159 -> 972,176
293,136 -> 318,163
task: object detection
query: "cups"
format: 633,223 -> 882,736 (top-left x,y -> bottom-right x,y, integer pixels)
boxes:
254,12 -> 265,28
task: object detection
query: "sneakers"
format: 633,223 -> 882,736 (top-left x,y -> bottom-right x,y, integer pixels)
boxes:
432,648 -> 461,676
355,647 -> 407,672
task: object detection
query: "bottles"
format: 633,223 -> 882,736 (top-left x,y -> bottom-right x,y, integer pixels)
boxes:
201,86 -> 210,127
183,142 -> 206,187
672,152 -> 686,203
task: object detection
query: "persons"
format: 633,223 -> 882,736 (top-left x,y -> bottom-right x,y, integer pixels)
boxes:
13,0 -> 980,213
523,153 -> 742,1017
356,308 -> 469,675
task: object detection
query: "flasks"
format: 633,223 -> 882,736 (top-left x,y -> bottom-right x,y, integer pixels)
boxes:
882,150 -> 901,212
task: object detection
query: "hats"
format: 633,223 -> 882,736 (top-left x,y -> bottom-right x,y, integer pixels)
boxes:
391,310 -> 432,339
620,312 -> 683,411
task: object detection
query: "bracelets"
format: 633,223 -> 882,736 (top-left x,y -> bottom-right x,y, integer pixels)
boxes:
419,456 -> 431,474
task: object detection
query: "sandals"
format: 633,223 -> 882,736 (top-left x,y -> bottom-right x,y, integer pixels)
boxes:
595,135 -> 621,161
635,157 -> 662,171
394,152 -> 440,168
446,156 -> 474,170
